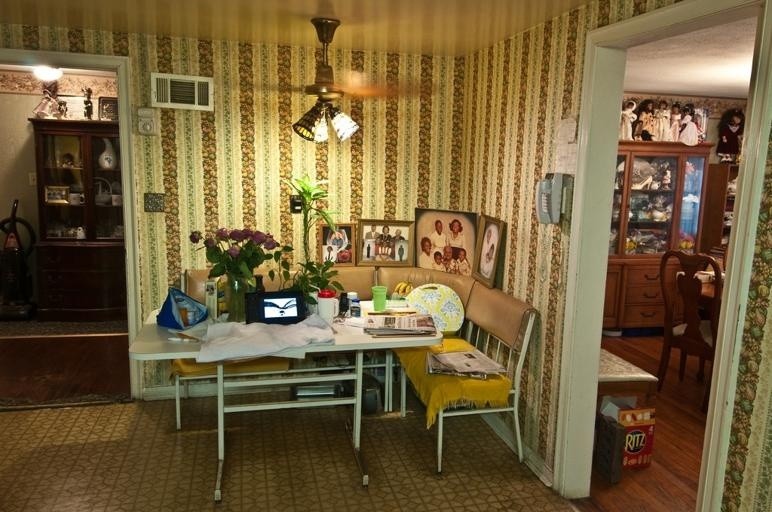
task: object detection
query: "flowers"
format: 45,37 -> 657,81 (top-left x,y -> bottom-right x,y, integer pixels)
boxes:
190,228 -> 280,322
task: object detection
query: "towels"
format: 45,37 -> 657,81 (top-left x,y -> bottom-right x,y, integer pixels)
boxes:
195,314 -> 336,365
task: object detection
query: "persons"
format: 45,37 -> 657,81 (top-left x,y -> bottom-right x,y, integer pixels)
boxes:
618,99 -> 703,146
325,230 -> 352,263
716,108 -> 745,164
419,218 -> 494,276
366,225 -> 405,262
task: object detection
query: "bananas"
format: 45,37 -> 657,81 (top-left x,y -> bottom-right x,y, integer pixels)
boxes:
394,281 -> 414,295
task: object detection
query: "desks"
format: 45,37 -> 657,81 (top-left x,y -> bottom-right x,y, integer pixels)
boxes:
700,277 -> 716,298
128,298 -> 444,504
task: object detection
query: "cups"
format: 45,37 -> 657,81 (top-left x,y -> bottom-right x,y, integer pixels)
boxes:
696,271 -> 714,284
371,286 -> 387,312
76,226 -> 87,239
69,193 -> 85,205
317,289 -> 340,326
114,225 -> 124,239
111,195 -> 122,206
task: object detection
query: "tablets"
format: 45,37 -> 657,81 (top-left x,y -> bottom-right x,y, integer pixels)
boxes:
258,294 -> 302,323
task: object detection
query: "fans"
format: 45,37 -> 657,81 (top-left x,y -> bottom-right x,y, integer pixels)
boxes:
242,79 -> 441,99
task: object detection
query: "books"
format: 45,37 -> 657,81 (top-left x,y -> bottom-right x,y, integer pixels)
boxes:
363,314 -> 437,337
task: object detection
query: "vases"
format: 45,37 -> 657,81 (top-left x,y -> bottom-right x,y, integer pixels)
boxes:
223,274 -> 251,323
98,138 -> 118,170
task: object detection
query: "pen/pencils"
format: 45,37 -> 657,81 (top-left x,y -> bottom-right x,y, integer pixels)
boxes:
368,313 -> 416,315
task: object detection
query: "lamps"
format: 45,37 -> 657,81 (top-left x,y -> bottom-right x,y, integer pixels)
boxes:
292,17 -> 360,143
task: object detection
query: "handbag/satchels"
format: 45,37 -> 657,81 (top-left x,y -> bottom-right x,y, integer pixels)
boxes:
156,286 -> 208,331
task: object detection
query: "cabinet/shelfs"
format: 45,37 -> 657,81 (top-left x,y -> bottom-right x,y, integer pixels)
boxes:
28,118 -> 124,241
37,248 -> 127,309
699,162 -> 739,254
602,140 -> 716,331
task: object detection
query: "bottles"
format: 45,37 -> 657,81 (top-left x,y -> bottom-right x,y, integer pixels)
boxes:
83,89 -> 94,120
340,292 -> 361,317
58,101 -> 67,117
217,270 -> 227,319
253,275 -> 265,293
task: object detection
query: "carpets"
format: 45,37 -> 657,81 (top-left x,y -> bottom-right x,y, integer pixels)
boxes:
0,319 -> 127,339
2,400 -> 580,511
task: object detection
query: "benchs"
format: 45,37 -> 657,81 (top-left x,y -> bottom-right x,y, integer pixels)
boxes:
593,347 -> 659,469
377,265 -> 540,473
173,262 -> 376,430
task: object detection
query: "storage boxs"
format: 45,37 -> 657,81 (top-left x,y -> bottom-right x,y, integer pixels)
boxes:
599,400 -> 656,471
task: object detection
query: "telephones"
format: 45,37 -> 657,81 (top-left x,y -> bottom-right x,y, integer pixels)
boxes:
536,172 -> 563,224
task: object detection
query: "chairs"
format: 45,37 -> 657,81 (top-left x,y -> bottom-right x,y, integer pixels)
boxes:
654,248 -> 722,413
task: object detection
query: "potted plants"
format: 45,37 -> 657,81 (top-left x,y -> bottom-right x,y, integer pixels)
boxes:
281,169 -> 344,313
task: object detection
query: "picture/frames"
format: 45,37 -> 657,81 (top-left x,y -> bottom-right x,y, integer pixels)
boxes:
99,96 -> 118,120
357,218 -> 416,267
314,221 -> 356,267
44,185 -> 70,204
470,213 -> 505,288
